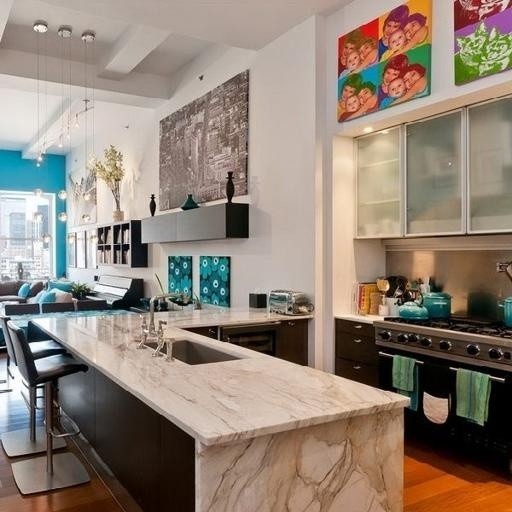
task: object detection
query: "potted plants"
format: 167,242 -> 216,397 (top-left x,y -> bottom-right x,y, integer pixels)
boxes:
89,144 -> 131,221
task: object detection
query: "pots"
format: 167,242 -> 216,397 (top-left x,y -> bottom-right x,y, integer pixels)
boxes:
497,296 -> 512,327
423,292 -> 453,318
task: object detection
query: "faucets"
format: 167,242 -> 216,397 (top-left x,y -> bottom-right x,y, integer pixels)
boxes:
139,293 -> 188,335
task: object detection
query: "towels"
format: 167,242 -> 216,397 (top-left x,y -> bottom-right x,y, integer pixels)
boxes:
390,355 -> 419,411
453,368 -> 491,426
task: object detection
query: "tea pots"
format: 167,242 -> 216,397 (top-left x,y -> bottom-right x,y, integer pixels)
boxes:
398,290 -> 429,319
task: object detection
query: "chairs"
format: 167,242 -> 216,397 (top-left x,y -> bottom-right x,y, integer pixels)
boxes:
5,320 -> 92,496
0,315 -> 68,458
42,302 -> 75,313
5,303 -> 40,379
76,299 -> 108,310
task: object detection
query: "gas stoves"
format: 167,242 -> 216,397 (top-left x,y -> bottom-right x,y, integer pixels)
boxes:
373,315 -> 512,373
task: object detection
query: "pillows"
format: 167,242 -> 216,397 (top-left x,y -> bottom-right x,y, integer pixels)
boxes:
18,283 -> 29,299
47,280 -> 73,293
34,290 -> 45,300
38,292 -> 54,307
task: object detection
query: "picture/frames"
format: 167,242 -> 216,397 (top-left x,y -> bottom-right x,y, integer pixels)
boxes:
336,1 -> 432,123
77,230 -> 86,269
453,0 -> 512,87
68,156 -> 97,230
158,68 -> 250,212
68,232 -> 76,269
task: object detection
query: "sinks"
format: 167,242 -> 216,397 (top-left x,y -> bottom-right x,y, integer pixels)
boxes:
147,340 -> 242,366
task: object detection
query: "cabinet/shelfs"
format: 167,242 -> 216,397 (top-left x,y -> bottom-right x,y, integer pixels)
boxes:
96,220 -> 148,268
141,202 -> 249,245
276,318 -> 308,367
353,120 -> 404,240
405,93 -> 512,236
335,317 -> 382,393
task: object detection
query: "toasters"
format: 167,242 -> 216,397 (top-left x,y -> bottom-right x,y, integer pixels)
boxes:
269,290 -> 314,316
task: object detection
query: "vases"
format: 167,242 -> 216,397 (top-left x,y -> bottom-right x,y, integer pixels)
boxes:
180,194 -> 199,210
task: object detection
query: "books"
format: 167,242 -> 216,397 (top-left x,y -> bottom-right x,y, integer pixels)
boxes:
356,282 -> 384,317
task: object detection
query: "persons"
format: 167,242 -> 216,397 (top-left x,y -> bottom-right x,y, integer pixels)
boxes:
378,54 -> 430,111
338,29 -> 378,79
338,73 -> 379,124
378,4 -> 428,64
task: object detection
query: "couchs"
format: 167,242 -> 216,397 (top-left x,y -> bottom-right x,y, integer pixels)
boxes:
0,280 -> 72,315
